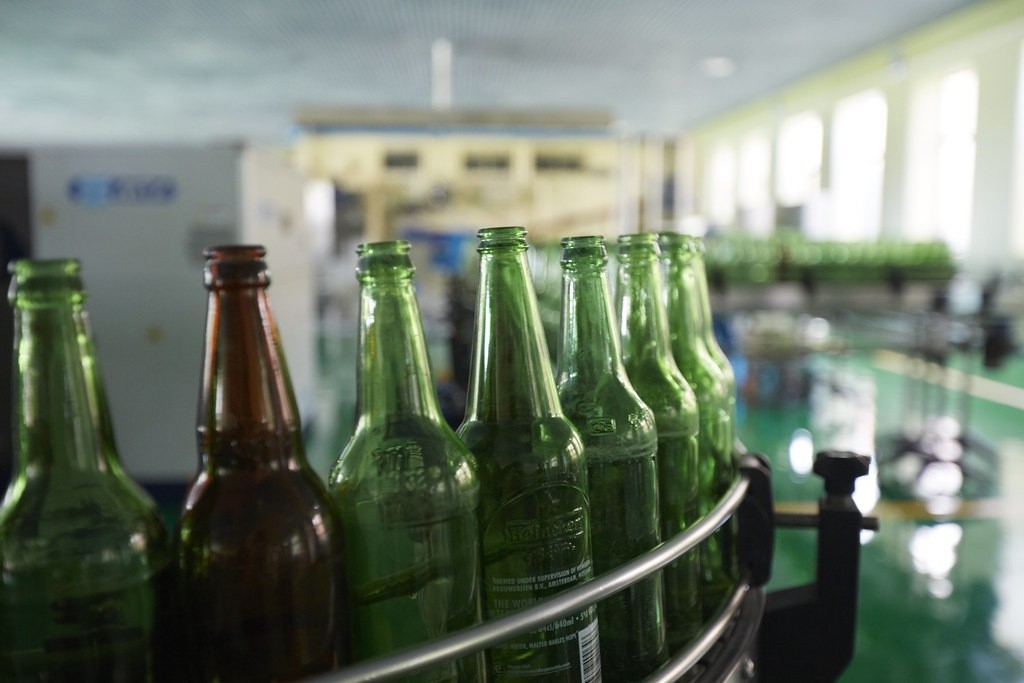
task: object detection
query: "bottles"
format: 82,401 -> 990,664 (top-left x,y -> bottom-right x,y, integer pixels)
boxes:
174,243 -> 341,683
556,234 -> 673,683
329,239 -> 487,683
618,228 -> 956,652
0,259 -> 175,682
453,226 -> 603,683
